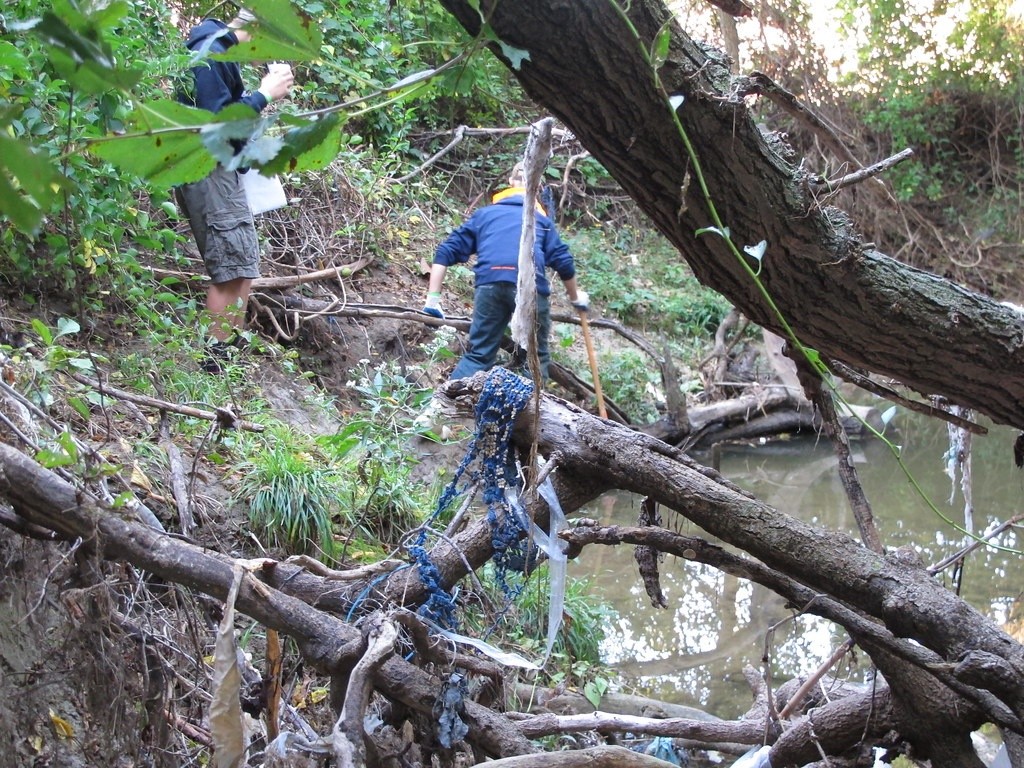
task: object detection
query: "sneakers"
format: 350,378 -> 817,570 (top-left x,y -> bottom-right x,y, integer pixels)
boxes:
200,329 -> 280,374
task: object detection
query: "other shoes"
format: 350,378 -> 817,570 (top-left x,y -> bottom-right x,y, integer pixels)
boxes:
413,414 -> 451,440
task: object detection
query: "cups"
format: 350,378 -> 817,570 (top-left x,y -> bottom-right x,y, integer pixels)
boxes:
267,61 -> 291,75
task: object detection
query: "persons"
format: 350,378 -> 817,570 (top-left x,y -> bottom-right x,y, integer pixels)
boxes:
413,163 -> 589,438
165,8 -> 295,372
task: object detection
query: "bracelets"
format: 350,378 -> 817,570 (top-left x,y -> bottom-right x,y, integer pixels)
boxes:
258,88 -> 272,103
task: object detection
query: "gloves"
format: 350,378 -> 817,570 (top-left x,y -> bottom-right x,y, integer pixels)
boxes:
422,292 -> 445,330
570,290 -> 592,311
